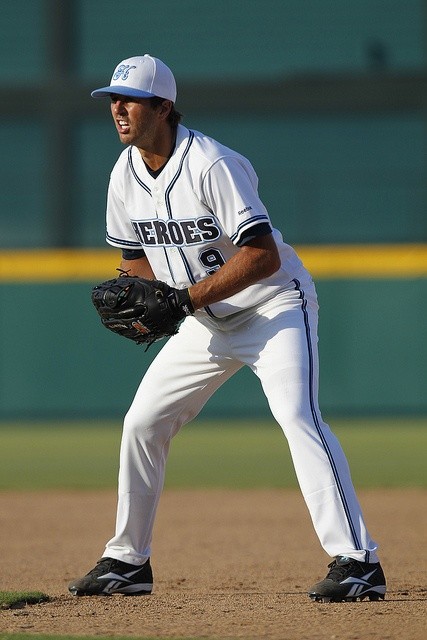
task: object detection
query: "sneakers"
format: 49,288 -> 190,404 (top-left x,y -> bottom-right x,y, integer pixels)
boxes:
68,556 -> 153,597
309,556 -> 387,603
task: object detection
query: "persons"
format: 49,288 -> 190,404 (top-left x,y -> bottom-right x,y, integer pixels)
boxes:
69,53 -> 387,601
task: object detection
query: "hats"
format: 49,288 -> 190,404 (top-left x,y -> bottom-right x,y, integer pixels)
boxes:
91,53 -> 177,106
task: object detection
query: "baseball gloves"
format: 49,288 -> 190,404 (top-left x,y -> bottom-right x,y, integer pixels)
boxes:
91,268 -> 194,353
